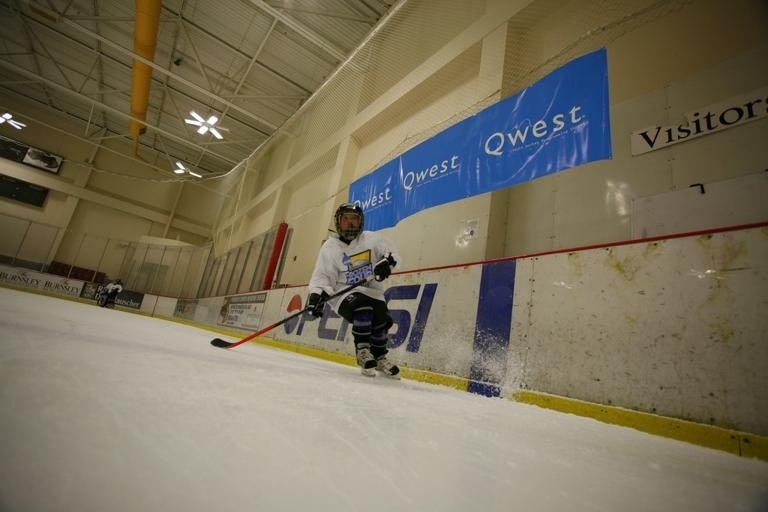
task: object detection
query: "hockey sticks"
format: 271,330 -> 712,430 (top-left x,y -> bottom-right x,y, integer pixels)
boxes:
211,276 -> 376,348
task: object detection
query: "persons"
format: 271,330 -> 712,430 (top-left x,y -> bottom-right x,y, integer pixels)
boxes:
102,278 -> 123,307
304,202 -> 400,377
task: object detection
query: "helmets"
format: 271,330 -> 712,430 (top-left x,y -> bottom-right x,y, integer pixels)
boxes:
333,203 -> 365,240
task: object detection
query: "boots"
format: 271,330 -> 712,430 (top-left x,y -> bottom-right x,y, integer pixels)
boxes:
354,343 -> 378,368
375,350 -> 400,376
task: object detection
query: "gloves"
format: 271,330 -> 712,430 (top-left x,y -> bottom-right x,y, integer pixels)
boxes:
306,294 -> 325,318
374,252 -> 398,283
